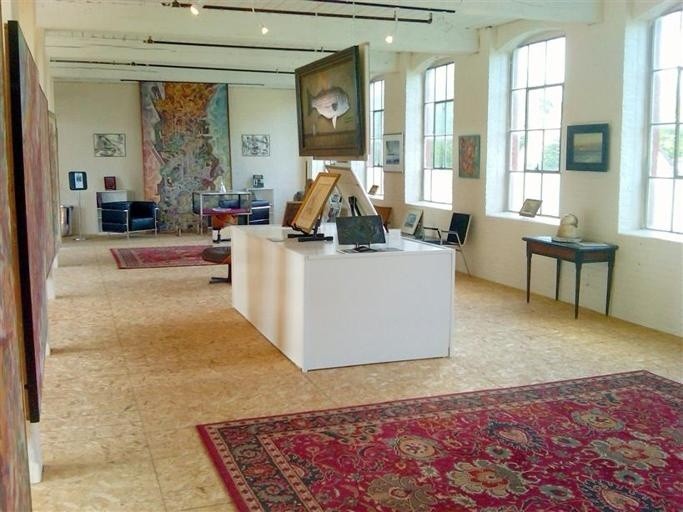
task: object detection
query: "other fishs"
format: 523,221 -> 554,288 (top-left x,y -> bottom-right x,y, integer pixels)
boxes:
306,86 -> 352,129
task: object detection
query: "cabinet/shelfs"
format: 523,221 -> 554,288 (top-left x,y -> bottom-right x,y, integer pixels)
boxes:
246,187 -> 276,225
96,190 -> 128,236
192,191 -> 252,235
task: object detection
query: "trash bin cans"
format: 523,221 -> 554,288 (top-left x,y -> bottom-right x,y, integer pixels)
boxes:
60,206 -> 73,236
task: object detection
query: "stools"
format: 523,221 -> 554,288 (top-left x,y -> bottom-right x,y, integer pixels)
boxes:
202,246 -> 231,284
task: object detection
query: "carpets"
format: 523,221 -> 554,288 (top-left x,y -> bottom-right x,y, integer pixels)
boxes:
195,369 -> 683,512
109,245 -> 227,269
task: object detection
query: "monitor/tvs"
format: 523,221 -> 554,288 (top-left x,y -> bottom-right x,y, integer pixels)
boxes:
335,215 -> 387,253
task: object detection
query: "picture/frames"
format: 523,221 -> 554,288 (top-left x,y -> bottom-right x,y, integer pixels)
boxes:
291,172 -> 342,233
295,45 -> 366,157
566,123 -> 609,172
104,176 -> 116,190
400,209 -> 423,235
519,199 -> 543,217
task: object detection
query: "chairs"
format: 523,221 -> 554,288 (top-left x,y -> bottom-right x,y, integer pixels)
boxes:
423,212 -> 473,279
101,201 -> 160,239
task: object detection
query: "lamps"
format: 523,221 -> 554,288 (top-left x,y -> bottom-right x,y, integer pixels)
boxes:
160,0 -> 432,44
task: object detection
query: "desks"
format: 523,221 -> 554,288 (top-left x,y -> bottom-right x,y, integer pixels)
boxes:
522,235 -> 619,319
230,222 -> 456,373
